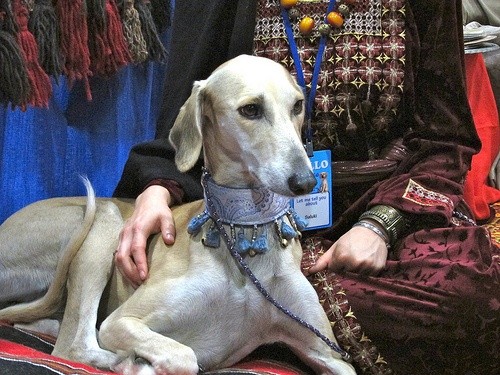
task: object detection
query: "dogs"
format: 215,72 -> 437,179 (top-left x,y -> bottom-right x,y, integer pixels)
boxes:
0,54 -> 359,374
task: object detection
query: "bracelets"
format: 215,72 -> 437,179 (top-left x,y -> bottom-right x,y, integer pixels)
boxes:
353,222 -> 389,246
358,204 -> 406,245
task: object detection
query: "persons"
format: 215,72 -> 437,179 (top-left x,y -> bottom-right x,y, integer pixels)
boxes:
112,0 -> 500,375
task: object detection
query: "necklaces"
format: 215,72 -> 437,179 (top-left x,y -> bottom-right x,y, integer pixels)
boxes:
280,0 -> 354,34
